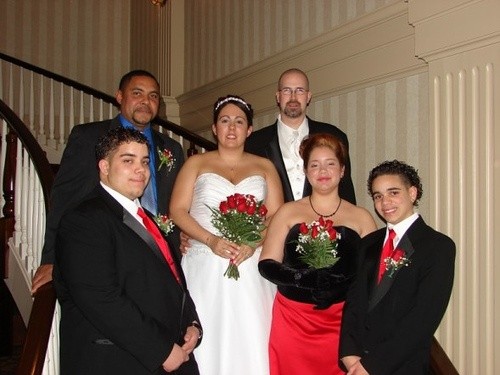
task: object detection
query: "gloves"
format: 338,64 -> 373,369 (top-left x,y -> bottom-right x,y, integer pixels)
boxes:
313,290 -> 356,309
258,259 -> 349,291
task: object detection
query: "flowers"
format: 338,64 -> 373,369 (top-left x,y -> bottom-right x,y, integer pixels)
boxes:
202,192 -> 268,281
158,148 -> 173,171
288,215 -> 342,270
384,248 -> 411,278
152,212 -> 175,236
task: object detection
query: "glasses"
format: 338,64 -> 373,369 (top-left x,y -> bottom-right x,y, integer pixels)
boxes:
278,88 -> 309,95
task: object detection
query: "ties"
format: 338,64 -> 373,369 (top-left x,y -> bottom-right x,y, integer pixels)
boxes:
140,130 -> 155,216
137,206 -> 183,289
293,131 -> 299,157
377,229 -> 396,285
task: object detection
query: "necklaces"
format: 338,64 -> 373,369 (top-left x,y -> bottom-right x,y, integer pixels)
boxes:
230,167 -> 234,171
309,194 -> 342,218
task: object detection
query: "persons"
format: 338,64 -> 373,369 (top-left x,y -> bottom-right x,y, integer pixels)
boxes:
339,159 -> 455,375
52,126 -> 204,375
258,133 -> 378,375
180,69 -> 357,256
32,69 -> 185,293
169,95 -> 284,375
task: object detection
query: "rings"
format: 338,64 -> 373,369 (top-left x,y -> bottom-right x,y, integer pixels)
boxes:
246,253 -> 249,257
242,251 -> 247,255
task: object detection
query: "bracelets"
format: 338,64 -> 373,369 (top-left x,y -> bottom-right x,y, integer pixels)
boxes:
206,234 -> 214,245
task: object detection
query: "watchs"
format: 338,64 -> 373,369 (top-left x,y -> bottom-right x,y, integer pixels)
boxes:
187,323 -> 203,338
